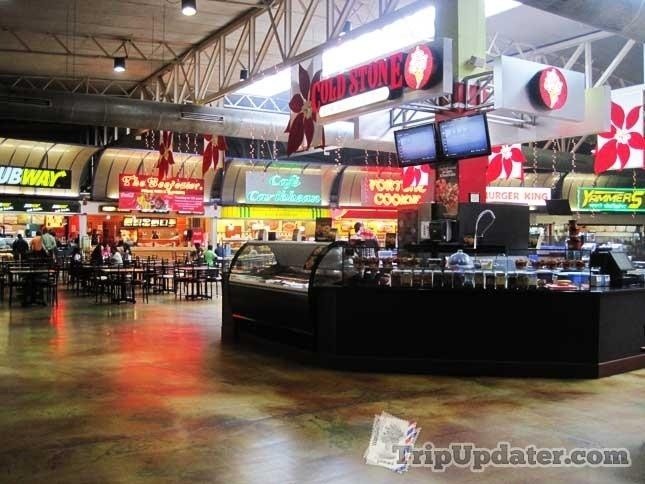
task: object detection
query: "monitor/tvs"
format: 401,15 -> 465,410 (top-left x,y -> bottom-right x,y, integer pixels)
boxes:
393,122 -> 437,167
435,111 -> 491,161
546,199 -> 572,215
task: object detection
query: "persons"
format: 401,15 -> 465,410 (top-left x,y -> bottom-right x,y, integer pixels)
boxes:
30,231 -> 43,257
90,245 -> 104,266
109,245 -> 123,267
190,243 -> 204,256
215,243 -> 225,257
72,246 -> 88,288
122,244 -> 132,264
153,232 -> 159,239
102,243 -> 112,262
12,234 -> 29,260
348,222 -> 381,270
91,229 -> 99,244
41,228 -> 56,257
203,244 -> 217,267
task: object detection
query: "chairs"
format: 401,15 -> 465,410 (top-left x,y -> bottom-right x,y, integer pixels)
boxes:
197,265 -> 217,298
93,263 -> 112,304
8,264 -> 27,306
44,264 -> 59,305
159,258 -> 177,293
136,261 -> 151,304
175,267 -> 196,298
66,258 -> 85,296
137,255 -> 152,297
29,263 -> 44,307
114,262 -> 136,304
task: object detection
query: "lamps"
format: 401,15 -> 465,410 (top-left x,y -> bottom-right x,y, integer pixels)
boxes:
113,57 -> 126,74
180,0 -> 196,15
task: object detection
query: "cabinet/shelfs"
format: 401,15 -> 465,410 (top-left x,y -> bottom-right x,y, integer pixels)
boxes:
344,247 -> 603,380
227,239 -> 336,367
601,248 -> 645,378
